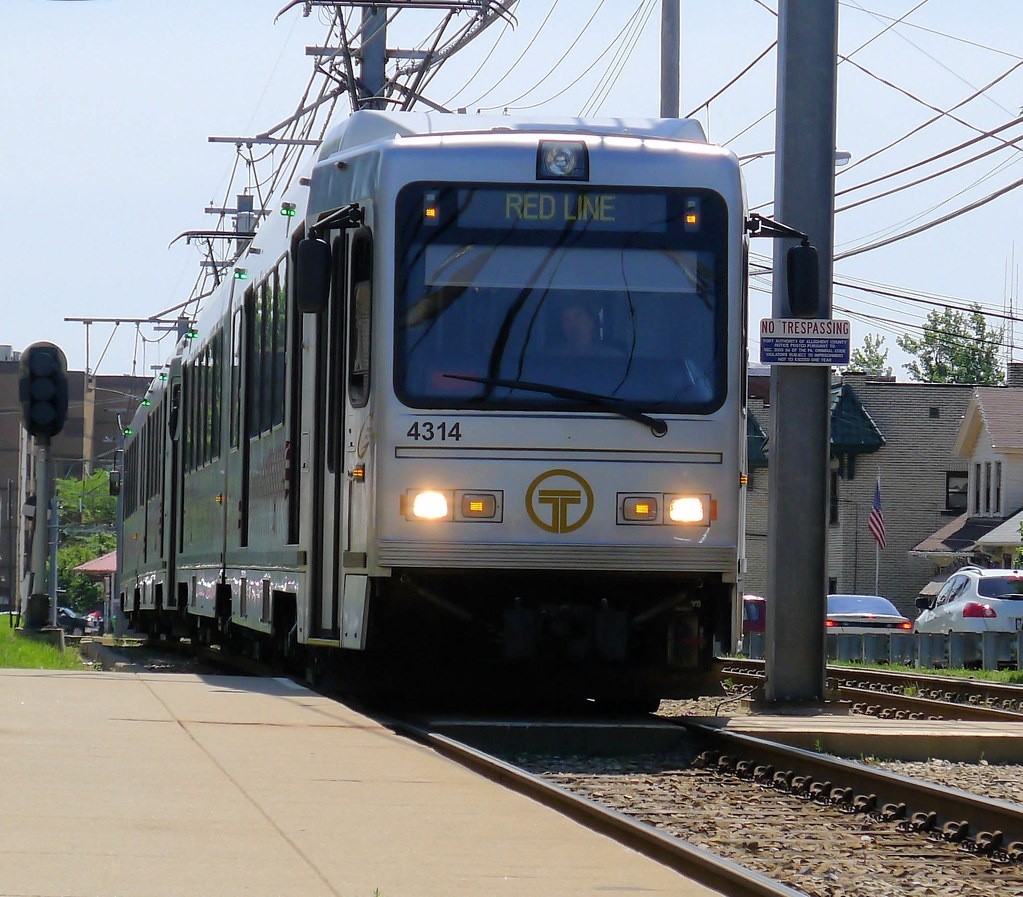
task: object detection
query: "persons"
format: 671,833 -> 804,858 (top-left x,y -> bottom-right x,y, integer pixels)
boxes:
546,302 -> 631,393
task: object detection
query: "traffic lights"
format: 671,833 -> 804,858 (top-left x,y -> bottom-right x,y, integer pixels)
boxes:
29,347 -> 62,437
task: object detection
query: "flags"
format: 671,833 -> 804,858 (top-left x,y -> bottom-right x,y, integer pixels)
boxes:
868,480 -> 888,549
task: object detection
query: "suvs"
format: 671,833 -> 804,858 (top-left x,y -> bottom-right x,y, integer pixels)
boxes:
912,566 -> 1023,632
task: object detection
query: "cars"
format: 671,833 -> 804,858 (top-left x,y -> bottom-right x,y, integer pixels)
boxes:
827,595 -> 912,634
57,607 -> 88,631
744,595 -> 766,634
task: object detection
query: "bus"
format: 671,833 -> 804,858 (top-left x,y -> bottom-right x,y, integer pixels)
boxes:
110,106 -> 825,724
